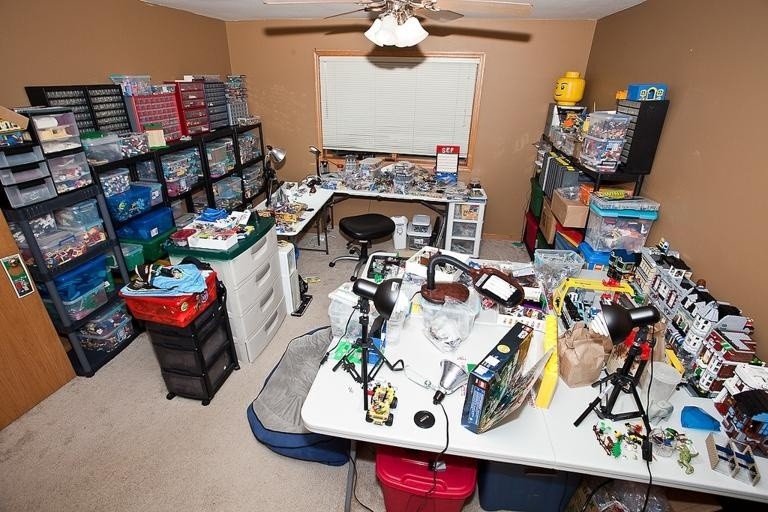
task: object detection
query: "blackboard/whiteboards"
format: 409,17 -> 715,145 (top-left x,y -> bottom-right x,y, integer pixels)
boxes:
313,49 -> 486,171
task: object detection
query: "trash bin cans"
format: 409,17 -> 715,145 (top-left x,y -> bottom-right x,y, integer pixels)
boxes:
390,215 -> 409,250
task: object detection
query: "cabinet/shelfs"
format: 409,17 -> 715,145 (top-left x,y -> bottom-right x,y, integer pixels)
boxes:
143,295 -> 241,409
160,213 -> 288,364
522,132 -> 646,260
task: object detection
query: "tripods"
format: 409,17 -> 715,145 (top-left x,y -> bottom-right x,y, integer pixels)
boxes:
332,338 -> 393,411
573,368 -> 665,432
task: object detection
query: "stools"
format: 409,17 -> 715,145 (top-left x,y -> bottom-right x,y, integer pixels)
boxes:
328,212 -> 396,282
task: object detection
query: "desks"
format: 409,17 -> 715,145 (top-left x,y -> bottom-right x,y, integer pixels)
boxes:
251,182 -> 334,271
301,174 -> 488,260
300,248 -> 767,512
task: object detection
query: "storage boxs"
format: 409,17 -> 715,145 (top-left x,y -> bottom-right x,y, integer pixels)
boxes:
406,222 -> 433,250
565,478 -> 724,512
476,456 -> 583,511
374,444 -> 477,510
411,213 -> 431,233
524,151 -> 660,264
532,247 -> 584,282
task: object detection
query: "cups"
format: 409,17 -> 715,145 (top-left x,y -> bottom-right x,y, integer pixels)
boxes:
639,362 -> 681,416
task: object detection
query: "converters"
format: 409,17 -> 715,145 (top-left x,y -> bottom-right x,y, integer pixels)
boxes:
642,441 -> 652,462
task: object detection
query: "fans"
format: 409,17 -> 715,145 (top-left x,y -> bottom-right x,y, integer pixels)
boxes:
261,1 -> 538,26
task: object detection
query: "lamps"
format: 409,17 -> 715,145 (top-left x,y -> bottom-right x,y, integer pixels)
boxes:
573,301 -> 661,437
262,141 -> 287,208
308,145 -> 324,182
351,276 -> 406,412
362,8 -> 429,51
421,252 -> 525,313
